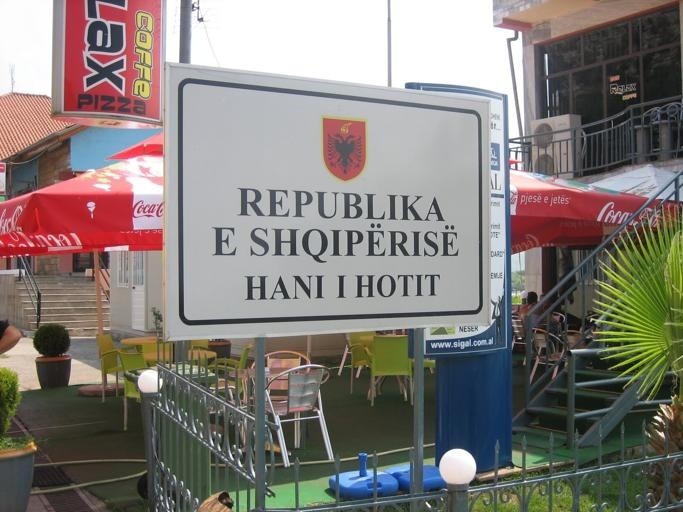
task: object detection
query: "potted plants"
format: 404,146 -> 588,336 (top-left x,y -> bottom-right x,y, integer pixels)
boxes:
0,367 -> 38,512
33,322 -> 71,388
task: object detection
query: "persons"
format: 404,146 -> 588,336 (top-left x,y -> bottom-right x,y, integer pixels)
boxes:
519,291 -> 546,355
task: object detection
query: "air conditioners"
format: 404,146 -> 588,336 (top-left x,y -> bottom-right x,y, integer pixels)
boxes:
530,114 -> 583,179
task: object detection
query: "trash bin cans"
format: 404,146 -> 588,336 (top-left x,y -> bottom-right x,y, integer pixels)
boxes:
124,363 -> 216,512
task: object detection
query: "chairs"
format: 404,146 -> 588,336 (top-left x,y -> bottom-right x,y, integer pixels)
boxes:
512,305 -> 582,384
337,330 -> 435,408
97,332 -> 333,467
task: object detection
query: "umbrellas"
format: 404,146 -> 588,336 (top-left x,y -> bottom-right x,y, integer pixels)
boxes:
508,163 -> 683,304
